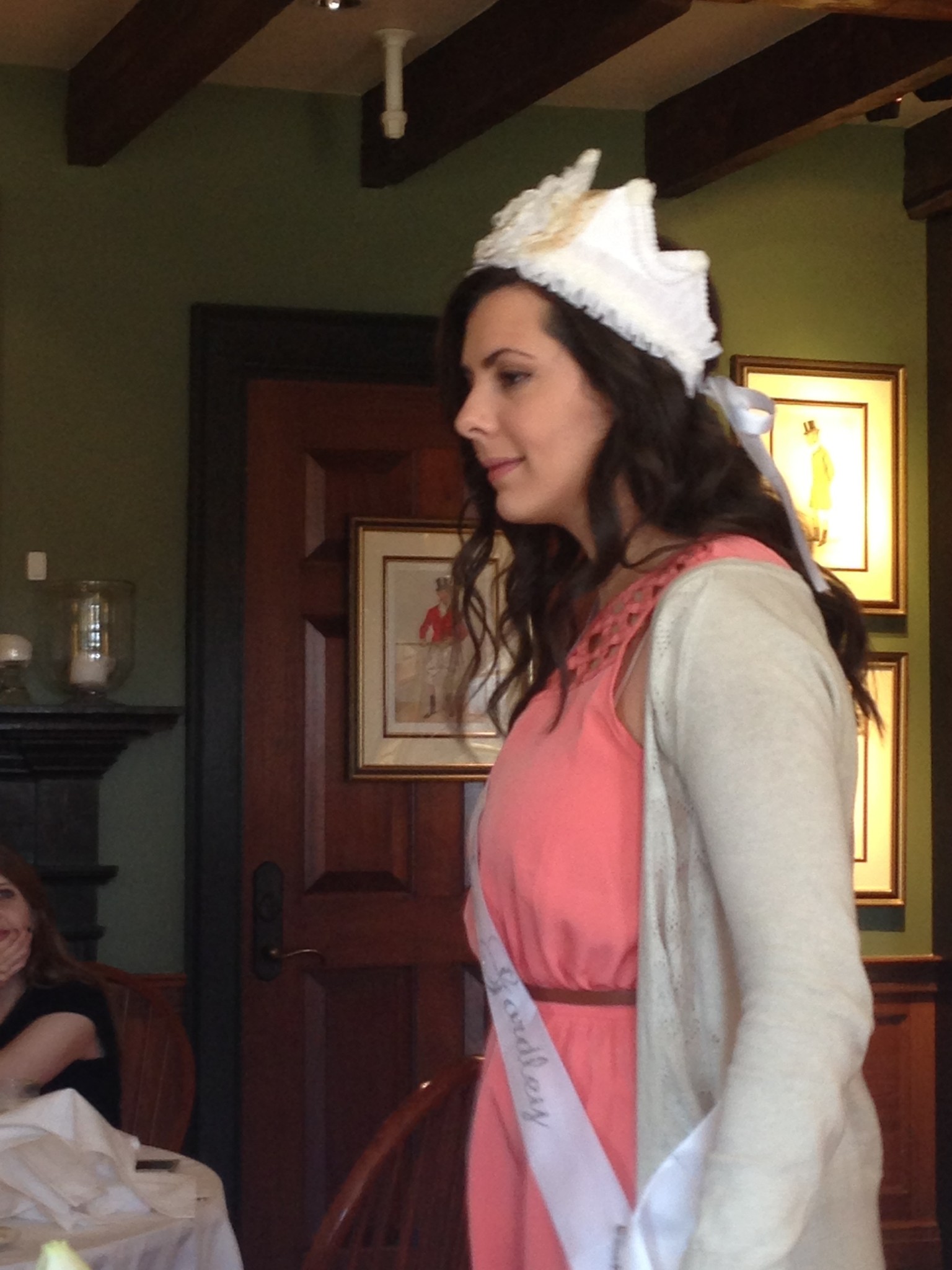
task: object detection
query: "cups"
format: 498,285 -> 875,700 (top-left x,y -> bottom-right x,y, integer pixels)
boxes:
51,577 -> 136,708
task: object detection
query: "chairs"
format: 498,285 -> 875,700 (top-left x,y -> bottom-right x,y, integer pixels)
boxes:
82,957 -> 197,1158
301,1052 -> 486,1270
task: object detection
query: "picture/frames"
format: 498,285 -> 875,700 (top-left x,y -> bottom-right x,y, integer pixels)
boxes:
730,350 -> 911,619
348,514 -> 513,781
850,648 -> 909,906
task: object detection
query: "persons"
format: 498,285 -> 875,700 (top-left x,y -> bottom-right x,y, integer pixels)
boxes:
1,841 -> 124,1129
452,146 -> 887,1270
